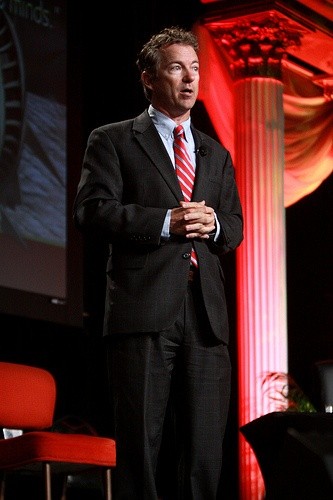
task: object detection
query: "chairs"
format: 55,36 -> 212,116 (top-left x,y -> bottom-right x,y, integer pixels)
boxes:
0,362 -> 116,500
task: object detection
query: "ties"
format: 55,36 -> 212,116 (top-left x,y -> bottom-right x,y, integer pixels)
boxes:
173,125 -> 198,269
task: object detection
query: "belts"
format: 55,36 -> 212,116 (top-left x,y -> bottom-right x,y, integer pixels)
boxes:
188,271 -> 198,281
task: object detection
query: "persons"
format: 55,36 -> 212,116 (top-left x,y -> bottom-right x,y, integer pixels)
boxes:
77,28 -> 244,500
